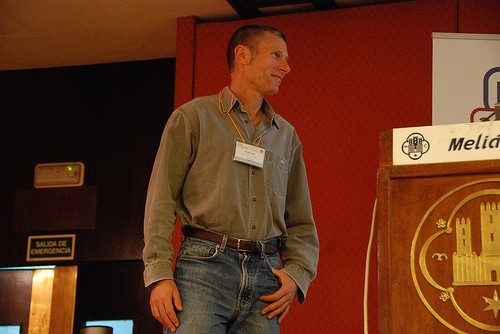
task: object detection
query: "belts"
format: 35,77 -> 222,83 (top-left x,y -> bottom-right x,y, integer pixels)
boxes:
183,226 -> 279,255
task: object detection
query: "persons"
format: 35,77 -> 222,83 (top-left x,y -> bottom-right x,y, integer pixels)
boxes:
141,24 -> 322,334
